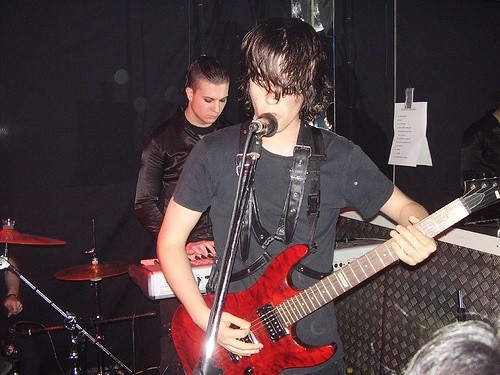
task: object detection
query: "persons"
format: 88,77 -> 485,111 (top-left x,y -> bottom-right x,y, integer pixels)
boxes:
134,54 -> 241,375
0,217 -> 24,318
404,311 -> 499,375
460,97 -> 500,222
156,18 -> 437,375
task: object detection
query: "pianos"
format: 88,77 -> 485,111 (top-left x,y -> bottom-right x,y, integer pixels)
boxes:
129,236 -> 389,301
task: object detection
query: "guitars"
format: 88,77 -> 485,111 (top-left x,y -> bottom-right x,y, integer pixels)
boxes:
167,173 -> 500,375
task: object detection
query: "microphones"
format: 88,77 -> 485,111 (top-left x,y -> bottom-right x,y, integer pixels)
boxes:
248,112 -> 278,137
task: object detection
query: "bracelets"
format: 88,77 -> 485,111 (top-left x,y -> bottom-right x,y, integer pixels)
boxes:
7,293 -> 19,299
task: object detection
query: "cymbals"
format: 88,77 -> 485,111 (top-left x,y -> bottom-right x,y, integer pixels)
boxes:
53,260 -> 129,281
0,228 -> 67,245
